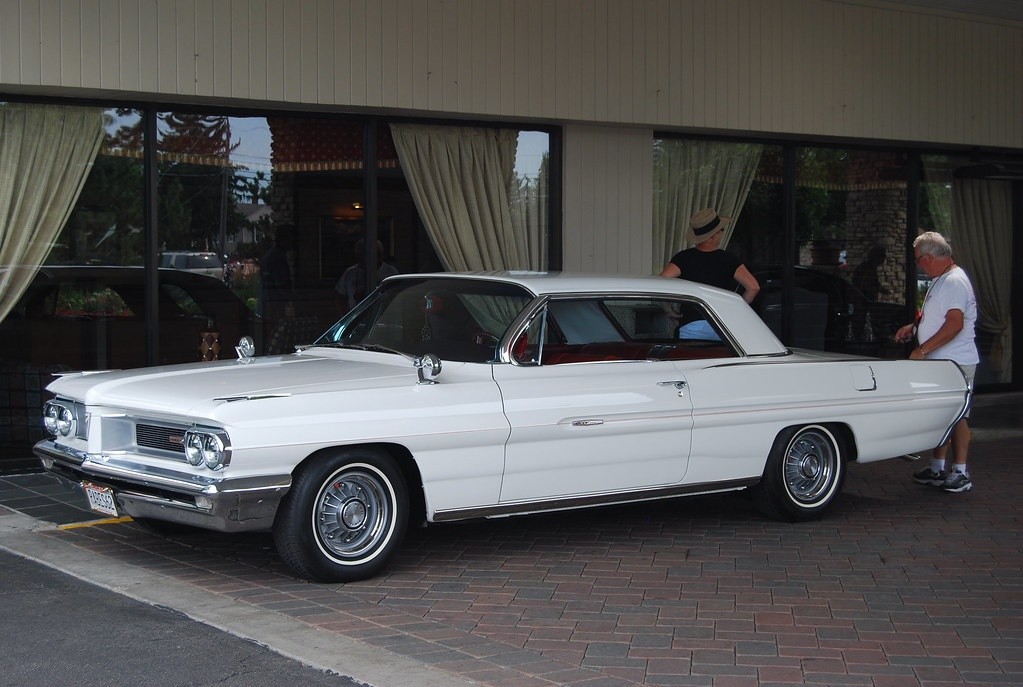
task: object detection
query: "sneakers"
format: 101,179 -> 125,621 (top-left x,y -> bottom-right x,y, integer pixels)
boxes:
912,463 -> 949,487
939,466 -> 972,493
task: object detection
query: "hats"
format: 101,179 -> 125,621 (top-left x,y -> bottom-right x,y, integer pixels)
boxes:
686,208 -> 732,245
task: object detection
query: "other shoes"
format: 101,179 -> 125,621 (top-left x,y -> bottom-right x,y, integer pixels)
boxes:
905,453 -> 921,460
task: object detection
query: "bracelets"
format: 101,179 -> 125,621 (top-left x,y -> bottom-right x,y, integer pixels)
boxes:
920,345 -> 929,355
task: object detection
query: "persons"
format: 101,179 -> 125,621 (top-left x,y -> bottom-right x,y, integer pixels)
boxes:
896,232 -> 980,492
158,240 -> 167,252
660,208 -> 759,340
266,295 -> 321,357
851,246 -> 891,342
336,241 -> 400,336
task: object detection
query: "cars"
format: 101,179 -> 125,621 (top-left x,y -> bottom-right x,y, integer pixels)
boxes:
32,269 -> 971,585
725,262 -> 918,357
0,260 -> 265,441
158,250 -> 246,289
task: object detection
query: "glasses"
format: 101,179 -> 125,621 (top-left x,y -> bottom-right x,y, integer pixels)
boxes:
914,253 -> 930,265
720,227 -> 724,231
